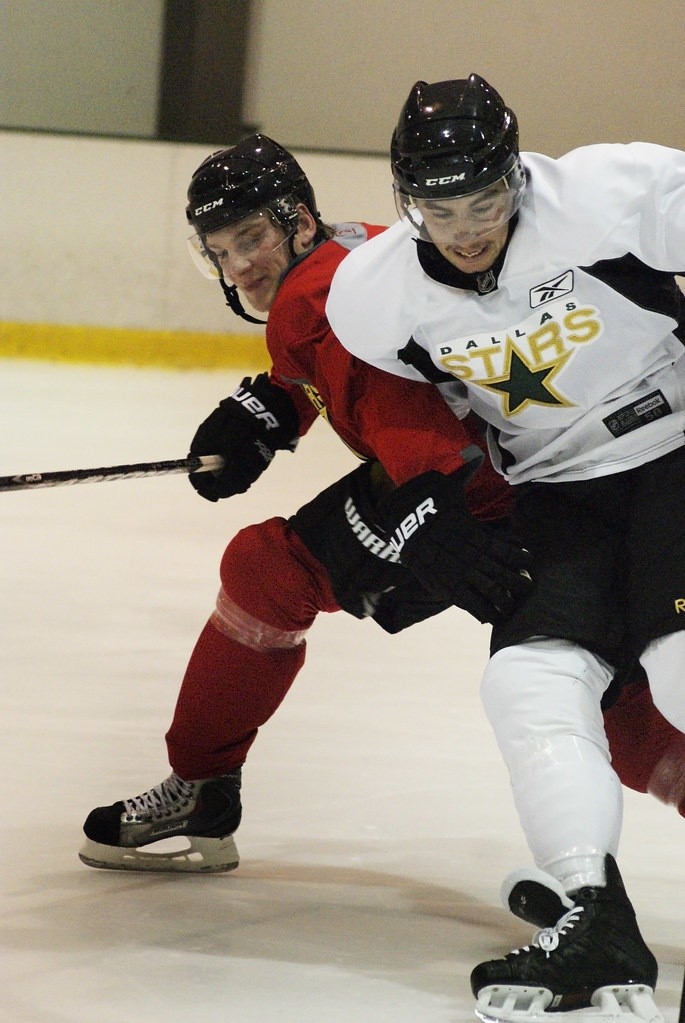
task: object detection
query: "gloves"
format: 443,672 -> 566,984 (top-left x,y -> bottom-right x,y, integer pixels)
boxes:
187,371 -> 302,503
312,469 -> 478,619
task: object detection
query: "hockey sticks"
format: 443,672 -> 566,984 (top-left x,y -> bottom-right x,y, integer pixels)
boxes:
2,456 -> 226,494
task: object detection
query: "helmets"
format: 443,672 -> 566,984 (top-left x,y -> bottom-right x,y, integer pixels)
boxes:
390,73 -> 525,242
185,134 -> 323,272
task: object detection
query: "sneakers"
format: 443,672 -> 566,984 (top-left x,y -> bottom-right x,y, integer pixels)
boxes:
78,764 -> 243,872
470,853 -> 664,1023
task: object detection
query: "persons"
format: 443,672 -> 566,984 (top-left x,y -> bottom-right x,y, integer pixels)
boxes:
325,74 -> 685,1023
78,133 -> 685,873
